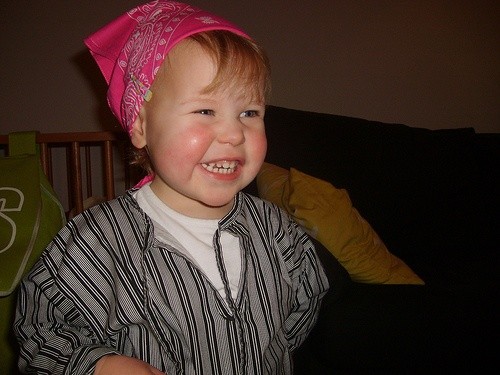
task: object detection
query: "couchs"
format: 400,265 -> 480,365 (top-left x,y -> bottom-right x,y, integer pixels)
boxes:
240,105 -> 500,375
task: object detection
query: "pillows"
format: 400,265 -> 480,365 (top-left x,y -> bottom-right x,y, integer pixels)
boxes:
257,162 -> 425,285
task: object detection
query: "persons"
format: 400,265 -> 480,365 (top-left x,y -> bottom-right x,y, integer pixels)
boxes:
12,0 -> 330,375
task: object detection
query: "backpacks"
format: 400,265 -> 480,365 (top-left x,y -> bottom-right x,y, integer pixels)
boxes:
0,124 -> 65,374
